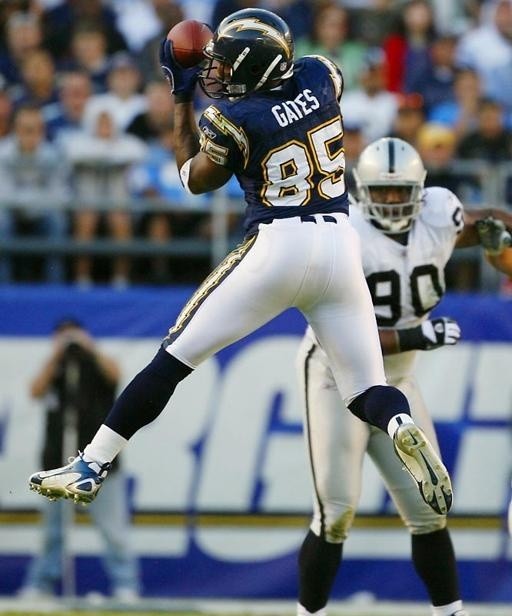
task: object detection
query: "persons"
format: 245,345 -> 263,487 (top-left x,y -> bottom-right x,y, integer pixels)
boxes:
25,5 -> 456,520
13,317 -> 146,604
294,135 -> 510,616
0,0 -> 512,291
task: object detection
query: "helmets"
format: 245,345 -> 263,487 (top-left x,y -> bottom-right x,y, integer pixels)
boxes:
353,136 -> 428,235
195,7 -> 295,99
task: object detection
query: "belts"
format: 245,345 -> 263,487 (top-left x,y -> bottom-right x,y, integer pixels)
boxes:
300,214 -> 338,223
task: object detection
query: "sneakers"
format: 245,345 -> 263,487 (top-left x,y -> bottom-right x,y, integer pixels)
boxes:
28,448 -> 114,506
393,422 -> 453,514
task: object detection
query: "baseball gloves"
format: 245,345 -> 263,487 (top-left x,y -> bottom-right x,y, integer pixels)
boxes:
477,218 -> 512,277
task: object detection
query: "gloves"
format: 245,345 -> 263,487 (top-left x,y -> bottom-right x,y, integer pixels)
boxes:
415,316 -> 462,350
474,216 -> 512,260
158,36 -> 208,94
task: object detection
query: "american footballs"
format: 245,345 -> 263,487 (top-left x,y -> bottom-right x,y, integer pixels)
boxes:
169,20 -> 213,69
